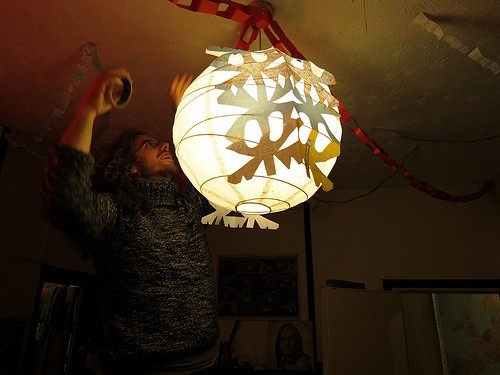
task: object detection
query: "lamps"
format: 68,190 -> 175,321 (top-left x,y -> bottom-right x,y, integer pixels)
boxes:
172,1 -> 345,229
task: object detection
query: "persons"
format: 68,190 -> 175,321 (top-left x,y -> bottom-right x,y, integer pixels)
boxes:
40,63 -> 236,375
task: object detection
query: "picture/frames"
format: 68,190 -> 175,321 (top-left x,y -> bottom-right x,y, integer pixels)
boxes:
211,253 -> 302,321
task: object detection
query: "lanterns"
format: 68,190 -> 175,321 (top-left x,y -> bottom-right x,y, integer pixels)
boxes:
172,46 -> 343,231
